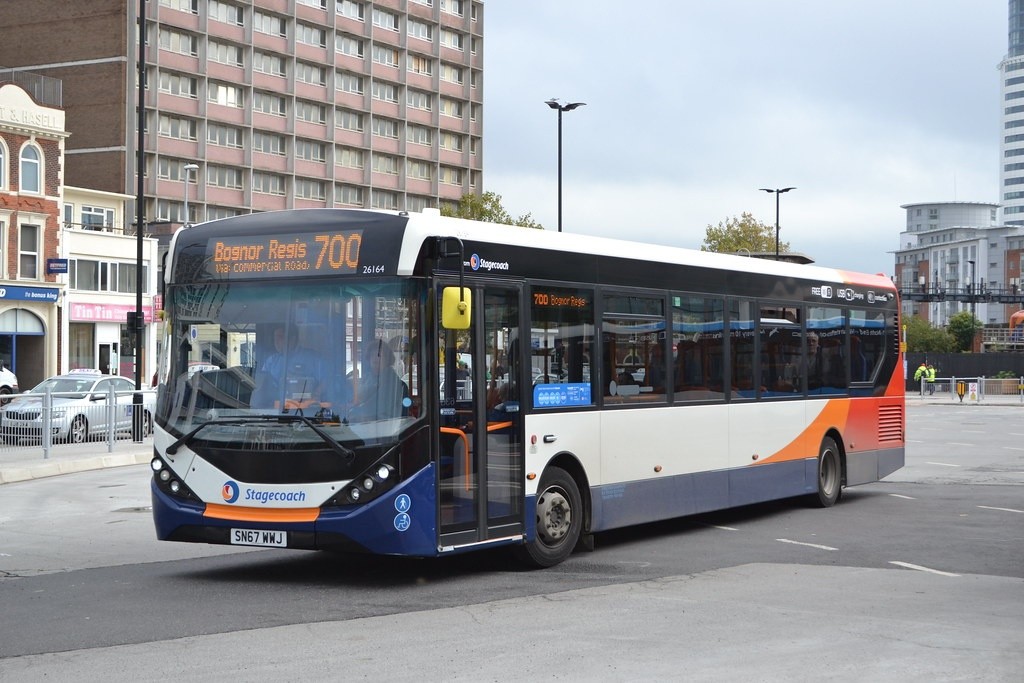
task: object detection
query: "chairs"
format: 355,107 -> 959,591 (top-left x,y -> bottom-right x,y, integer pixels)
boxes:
616,328 -> 877,390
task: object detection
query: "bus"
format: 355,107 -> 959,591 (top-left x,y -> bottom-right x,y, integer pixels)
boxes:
150,207 -> 907,569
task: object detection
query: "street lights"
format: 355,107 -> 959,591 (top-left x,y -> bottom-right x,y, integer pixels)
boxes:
758,188 -> 796,261
966,260 -> 976,353
545,102 -> 587,233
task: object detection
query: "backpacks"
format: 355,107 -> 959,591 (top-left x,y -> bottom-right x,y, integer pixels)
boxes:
918,369 -> 926,380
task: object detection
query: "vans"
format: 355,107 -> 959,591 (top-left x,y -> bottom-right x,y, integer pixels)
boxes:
0,360 -> 19,407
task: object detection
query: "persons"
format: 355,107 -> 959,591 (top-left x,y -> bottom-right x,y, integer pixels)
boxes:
250,322 -> 329,410
617,329 -> 864,392
498,383 -> 520,403
913,362 -> 936,396
343,341 -> 408,423
455,359 -> 504,399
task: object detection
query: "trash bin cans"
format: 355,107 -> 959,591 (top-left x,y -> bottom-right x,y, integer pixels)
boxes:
957,381 -> 965,402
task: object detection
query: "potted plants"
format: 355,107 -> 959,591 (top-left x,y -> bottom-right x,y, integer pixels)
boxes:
982,369 -> 1018,394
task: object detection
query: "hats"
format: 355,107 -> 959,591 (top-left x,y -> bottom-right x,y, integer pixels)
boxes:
459,360 -> 465,365
366,339 -> 394,366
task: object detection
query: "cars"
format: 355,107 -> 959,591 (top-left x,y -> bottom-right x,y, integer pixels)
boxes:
0,369 -> 156,443
